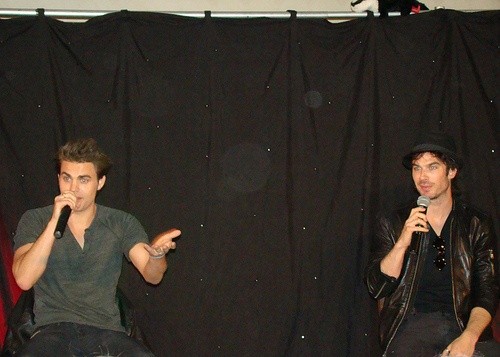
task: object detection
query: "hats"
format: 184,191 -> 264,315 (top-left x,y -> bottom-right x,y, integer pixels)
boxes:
401,132 -> 464,170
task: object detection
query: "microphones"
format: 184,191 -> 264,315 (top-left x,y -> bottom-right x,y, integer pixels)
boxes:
409,196 -> 431,255
53,205 -> 72,239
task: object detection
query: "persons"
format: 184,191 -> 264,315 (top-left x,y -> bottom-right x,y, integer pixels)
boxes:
12,139 -> 183,356
366,133 -> 497,357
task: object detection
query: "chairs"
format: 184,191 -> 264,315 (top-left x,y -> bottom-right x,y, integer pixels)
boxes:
2,285 -> 155,357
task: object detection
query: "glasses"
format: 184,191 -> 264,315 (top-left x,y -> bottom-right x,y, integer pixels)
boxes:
433,236 -> 448,272
